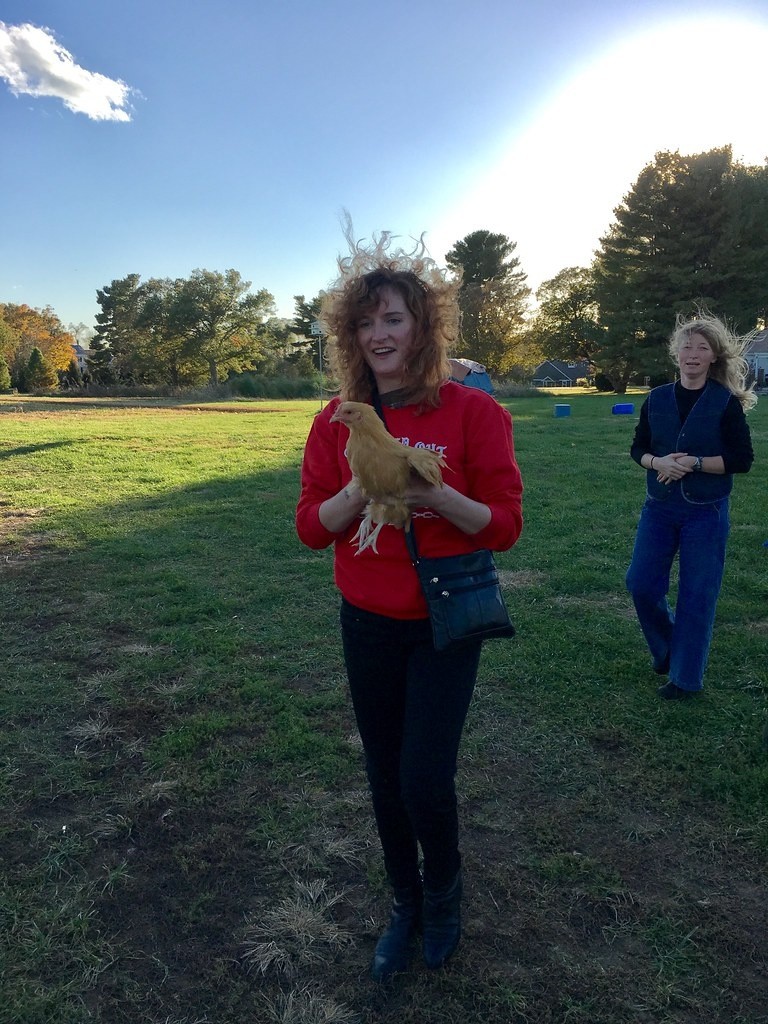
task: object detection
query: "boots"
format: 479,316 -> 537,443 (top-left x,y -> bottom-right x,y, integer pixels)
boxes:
420,871 -> 462,971
373,894 -> 422,973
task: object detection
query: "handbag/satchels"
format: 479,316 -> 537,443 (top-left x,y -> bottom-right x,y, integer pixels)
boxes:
411,547 -> 516,649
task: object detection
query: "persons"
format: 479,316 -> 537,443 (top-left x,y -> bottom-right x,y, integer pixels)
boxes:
626,320 -> 754,702
293,253 -> 526,975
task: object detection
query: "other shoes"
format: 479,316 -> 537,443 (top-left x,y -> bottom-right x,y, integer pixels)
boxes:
652,649 -> 670,675
657,681 -> 699,699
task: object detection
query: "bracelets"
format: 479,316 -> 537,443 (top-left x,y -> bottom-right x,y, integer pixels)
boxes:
693,455 -> 704,473
651,456 -> 656,470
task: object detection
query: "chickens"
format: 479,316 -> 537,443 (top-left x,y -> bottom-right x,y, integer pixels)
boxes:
328,402 -> 455,556
